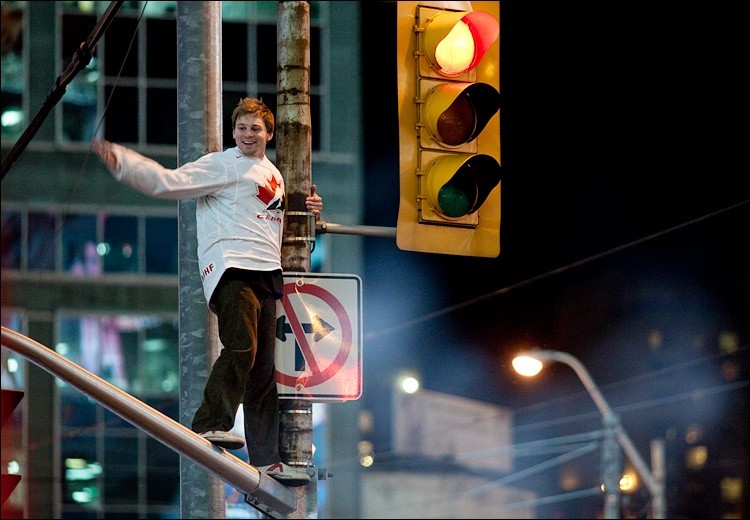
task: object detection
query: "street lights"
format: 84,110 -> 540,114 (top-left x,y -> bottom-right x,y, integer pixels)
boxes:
510,348 -> 671,520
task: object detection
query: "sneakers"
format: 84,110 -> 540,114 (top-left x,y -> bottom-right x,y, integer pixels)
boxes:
196,431 -> 245,450
256,462 -> 310,486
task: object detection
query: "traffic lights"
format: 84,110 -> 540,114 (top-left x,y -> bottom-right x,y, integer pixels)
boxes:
396,0 -> 503,259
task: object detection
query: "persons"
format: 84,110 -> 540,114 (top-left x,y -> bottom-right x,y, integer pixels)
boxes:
86,99 -> 323,487
